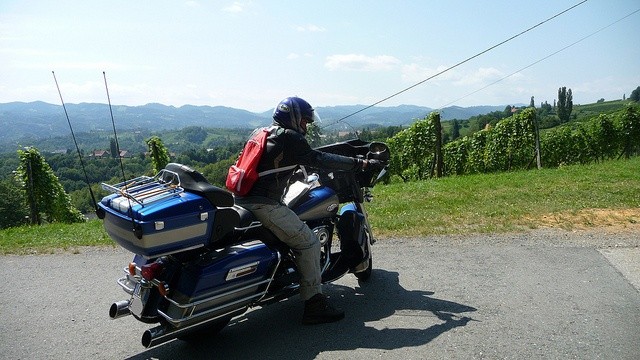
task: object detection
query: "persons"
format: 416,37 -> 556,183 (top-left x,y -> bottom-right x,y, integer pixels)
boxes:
233,97 -> 384,326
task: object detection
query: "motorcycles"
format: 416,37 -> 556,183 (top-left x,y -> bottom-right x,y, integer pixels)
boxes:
52,69 -> 392,349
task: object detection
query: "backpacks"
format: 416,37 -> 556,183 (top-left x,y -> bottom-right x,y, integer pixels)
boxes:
225,126 -> 299,196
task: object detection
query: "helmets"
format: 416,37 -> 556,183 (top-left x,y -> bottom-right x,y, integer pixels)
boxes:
273,96 -> 322,136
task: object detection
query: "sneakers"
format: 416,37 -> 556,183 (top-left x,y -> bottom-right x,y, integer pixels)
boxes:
301,293 -> 345,326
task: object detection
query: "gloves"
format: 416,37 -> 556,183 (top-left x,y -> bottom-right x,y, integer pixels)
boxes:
363,158 -> 385,172
